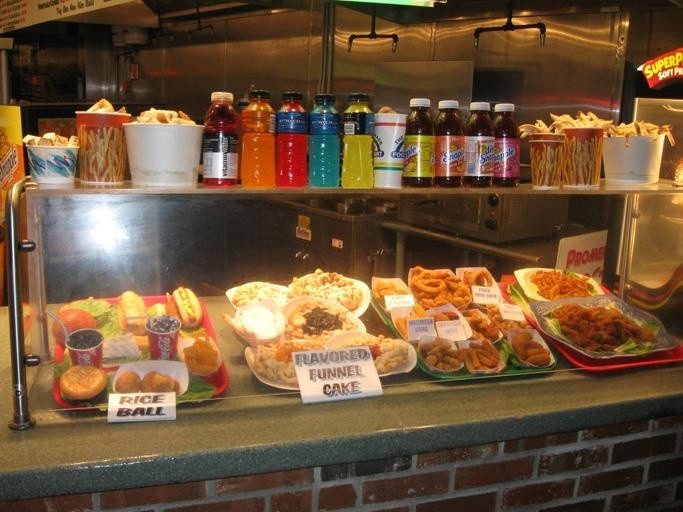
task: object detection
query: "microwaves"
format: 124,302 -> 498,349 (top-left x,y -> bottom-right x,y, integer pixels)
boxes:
393,186 -> 572,245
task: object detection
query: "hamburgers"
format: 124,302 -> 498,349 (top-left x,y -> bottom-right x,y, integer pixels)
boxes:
59,366 -> 109,406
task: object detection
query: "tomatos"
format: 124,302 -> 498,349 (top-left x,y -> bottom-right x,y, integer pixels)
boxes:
53,309 -> 97,343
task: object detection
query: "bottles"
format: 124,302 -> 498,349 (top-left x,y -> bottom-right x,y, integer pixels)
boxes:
202,88 -> 240,187
492,104 -> 520,187
464,101 -> 496,187
405,98 -> 436,185
275,89 -> 309,187
343,93 -> 374,187
434,100 -> 464,187
308,93 -> 341,188
240,89 -> 276,186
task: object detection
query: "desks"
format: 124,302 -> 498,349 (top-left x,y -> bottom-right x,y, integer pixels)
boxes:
382,217 -> 557,279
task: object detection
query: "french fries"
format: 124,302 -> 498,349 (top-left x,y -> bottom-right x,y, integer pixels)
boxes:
463,339 -> 501,372
519,111 -> 675,148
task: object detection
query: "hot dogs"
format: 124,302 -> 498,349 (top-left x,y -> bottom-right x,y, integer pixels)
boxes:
172,283 -> 203,328
118,289 -> 147,334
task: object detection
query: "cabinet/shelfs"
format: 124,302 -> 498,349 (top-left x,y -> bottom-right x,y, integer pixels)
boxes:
257,201 -> 431,290
0,179 -> 682,512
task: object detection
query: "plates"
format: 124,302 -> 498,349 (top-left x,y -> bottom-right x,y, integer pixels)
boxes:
113,335 -> 220,398
499,267 -> 682,375
225,267 -> 417,390
370,267 -> 558,379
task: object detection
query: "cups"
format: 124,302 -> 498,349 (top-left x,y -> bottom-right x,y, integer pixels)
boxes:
66,330 -> 104,367
23,144 -> 81,185
600,133 -> 666,184
119,119 -> 205,188
560,128 -> 606,191
528,132 -> 564,191
145,315 -> 181,359
73,109 -> 132,187
372,110 -> 409,187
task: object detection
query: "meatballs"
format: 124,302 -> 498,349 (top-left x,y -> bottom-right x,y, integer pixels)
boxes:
116,372 -> 179,398
